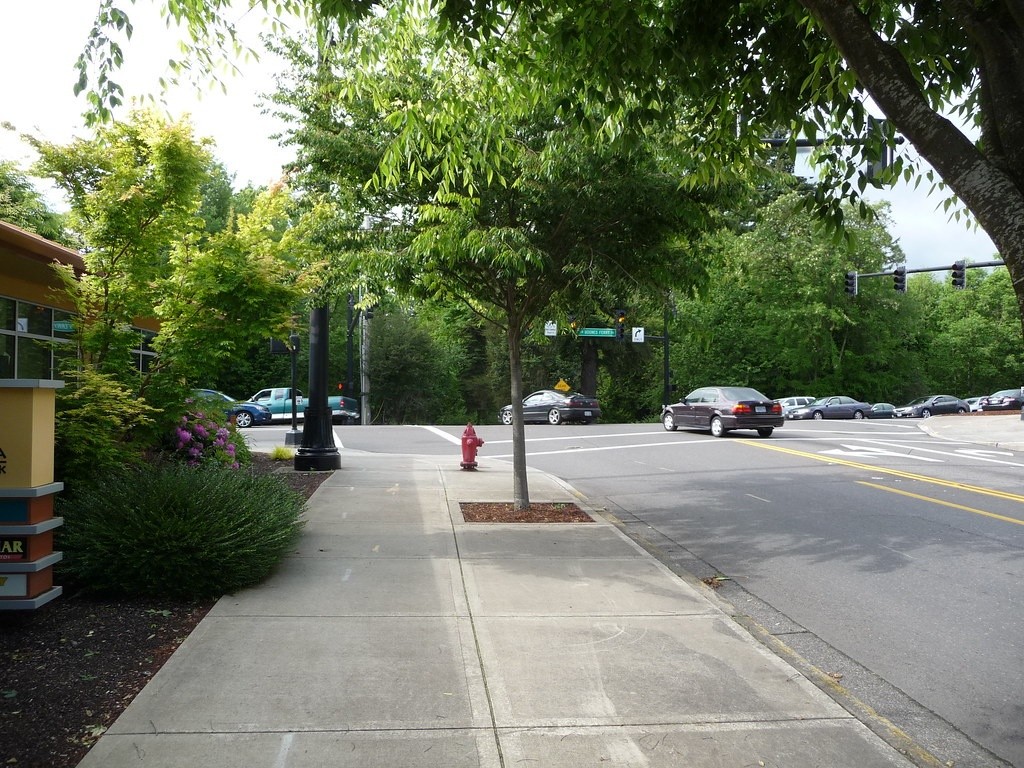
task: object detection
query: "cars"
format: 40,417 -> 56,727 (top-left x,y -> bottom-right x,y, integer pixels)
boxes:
869,403 -> 896,419
893,394 -> 970,418
774,397 -> 816,419
190,387 -> 272,428
788,396 -> 872,420
660,386 -> 785,437
963,388 -> 1024,412
498,390 -> 602,425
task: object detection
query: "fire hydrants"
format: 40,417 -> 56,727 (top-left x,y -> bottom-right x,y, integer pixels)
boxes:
460,422 -> 484,471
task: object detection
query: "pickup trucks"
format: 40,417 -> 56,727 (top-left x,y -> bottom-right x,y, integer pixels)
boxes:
247,388 -> 360,424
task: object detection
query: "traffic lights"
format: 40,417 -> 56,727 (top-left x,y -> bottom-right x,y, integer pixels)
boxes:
845,274 -> 855,293
615,311 -> 624,340
569,316 -> 577,329
952,263 -> 965,286
894,269 -> 905,290
